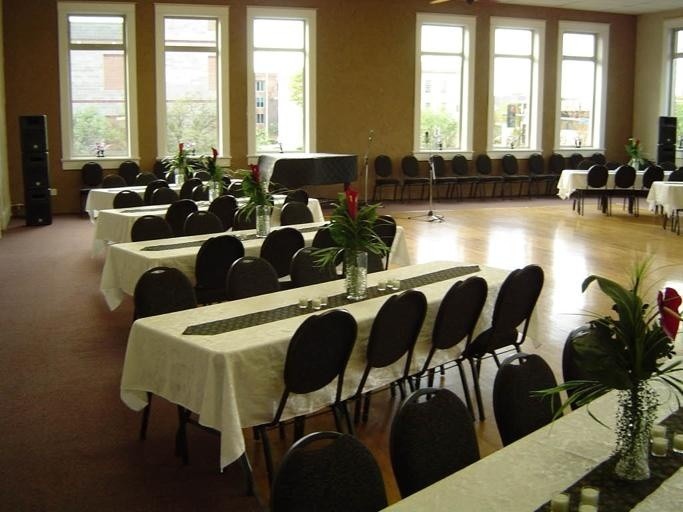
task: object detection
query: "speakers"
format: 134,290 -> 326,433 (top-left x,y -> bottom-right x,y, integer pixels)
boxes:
659,117 -> 677,167
18,116 -> 54,226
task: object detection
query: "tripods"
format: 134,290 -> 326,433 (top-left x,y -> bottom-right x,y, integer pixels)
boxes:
407,161 -> 445,222
359,142 -> 384,211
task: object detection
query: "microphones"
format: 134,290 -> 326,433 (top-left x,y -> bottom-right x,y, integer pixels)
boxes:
369,128 -> 374,143
423,131 -> 430,143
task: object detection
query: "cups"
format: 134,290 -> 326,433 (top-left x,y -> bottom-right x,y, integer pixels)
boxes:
580,488 -> 599,510
672,434 -> 683,453
320,295 -> 328,306
391,279 -> 401,291
376,279 -> 386,291
648,424 -> 666,437
547,491 -> 572,512
312,297 -> 321,308
651,437 -> 668,459
298,296 -> 307,310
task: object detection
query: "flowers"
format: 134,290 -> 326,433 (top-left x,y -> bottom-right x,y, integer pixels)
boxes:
623,137 -> 643,163
160,142 -> 197,178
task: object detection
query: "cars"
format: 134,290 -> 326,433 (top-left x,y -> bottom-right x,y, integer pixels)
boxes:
559,116 -> 590,147
